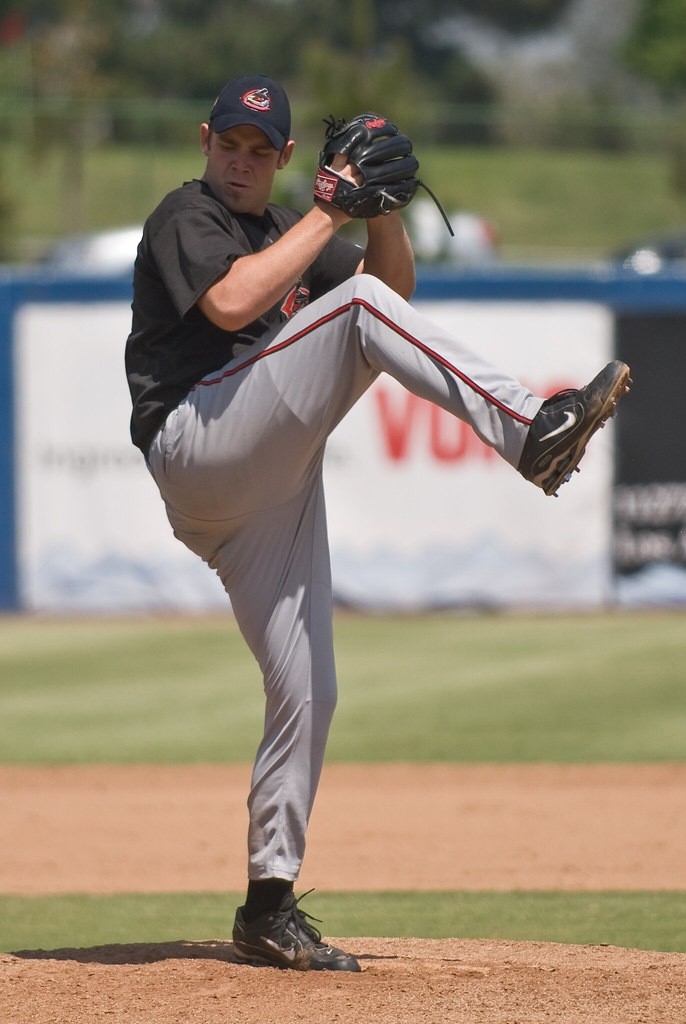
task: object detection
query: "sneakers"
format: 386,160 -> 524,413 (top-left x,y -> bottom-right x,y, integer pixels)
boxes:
517,360 -> 634,498
231,888 -> 361,972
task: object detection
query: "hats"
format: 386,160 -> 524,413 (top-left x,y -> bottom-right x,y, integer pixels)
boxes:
211,75 -> 290,150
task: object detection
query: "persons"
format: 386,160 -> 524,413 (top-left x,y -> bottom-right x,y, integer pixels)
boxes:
123,75 -> 633,977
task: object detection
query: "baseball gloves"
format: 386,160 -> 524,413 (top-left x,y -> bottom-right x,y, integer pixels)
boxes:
311,117 -> 420,220
